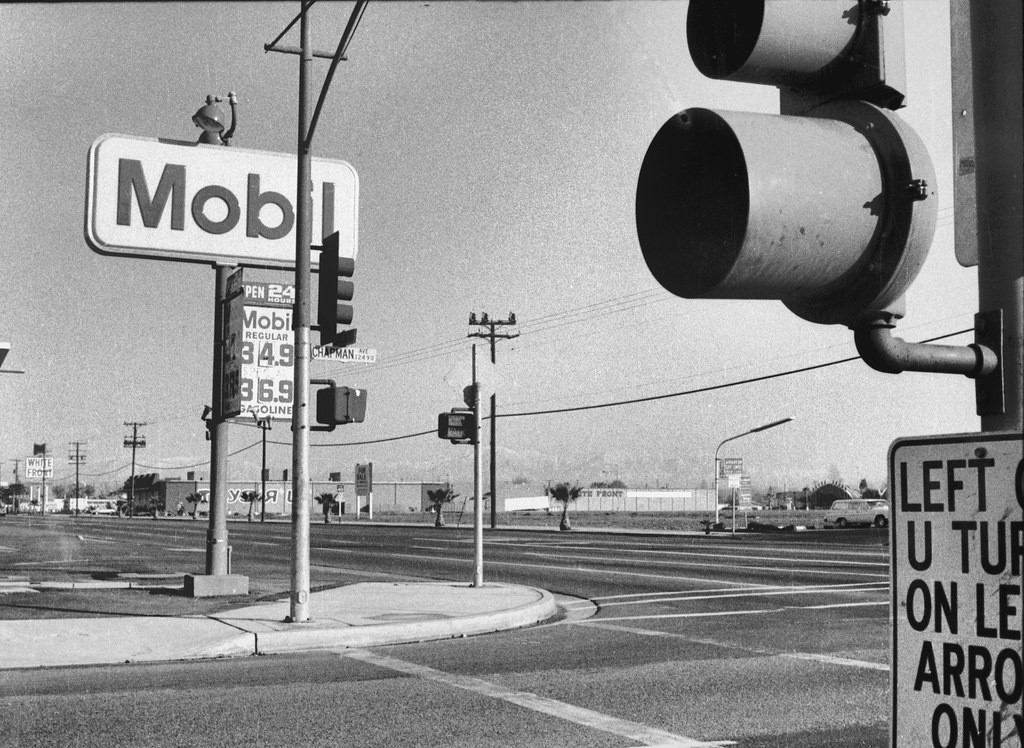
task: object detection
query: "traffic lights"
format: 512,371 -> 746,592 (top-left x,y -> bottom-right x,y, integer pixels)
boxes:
318,230 -> 355,349
316,385 -> 368,425
437,407 -> 476,446
632,0 -> 1008,418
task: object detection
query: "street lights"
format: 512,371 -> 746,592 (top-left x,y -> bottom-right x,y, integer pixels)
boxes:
713,416 -> 797,528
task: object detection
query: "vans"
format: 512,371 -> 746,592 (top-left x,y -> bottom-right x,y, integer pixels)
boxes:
824,498 -> 889,528
